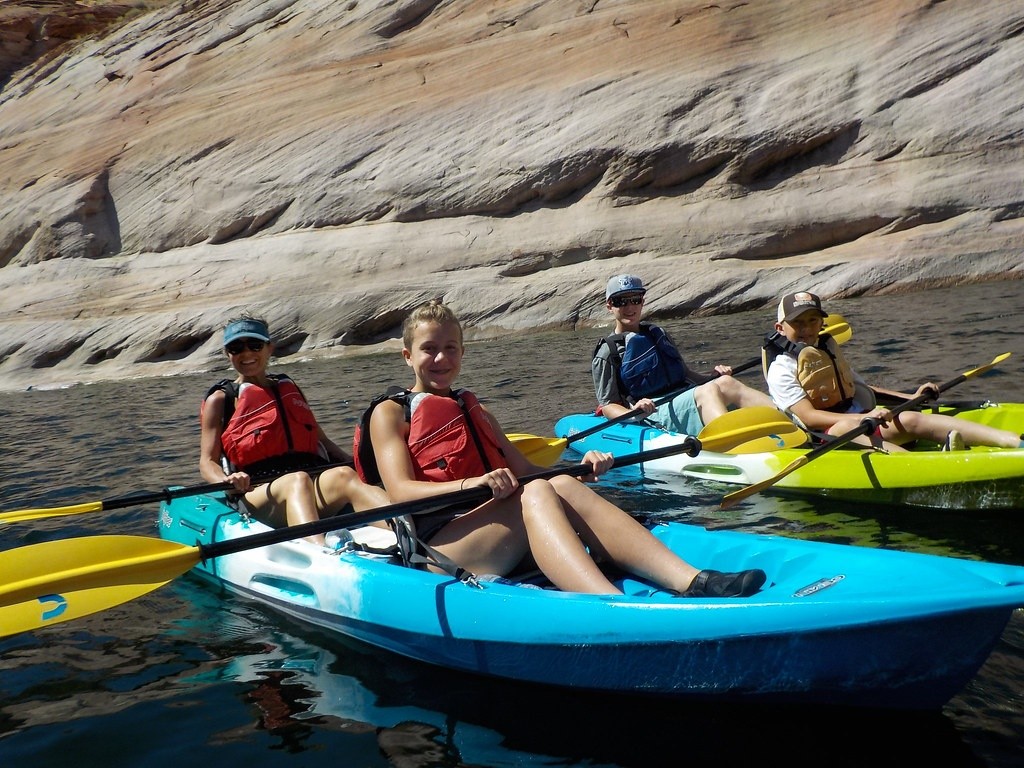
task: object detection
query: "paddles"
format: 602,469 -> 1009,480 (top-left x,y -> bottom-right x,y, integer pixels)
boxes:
0,405 -> 808,640
508,312 -> 853,467
0,431 -> 539,528
718,351 -> 1013,509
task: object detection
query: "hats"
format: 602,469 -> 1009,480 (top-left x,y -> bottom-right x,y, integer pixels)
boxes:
606,274 -> 646,303
777,291 -> 828,324
223,320 -> 270,350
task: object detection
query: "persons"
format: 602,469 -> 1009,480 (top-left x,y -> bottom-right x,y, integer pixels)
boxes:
592,274 -> 776,437
200,313 -> 395,546
767,291 -> 1024,453
370,301 -> 767,597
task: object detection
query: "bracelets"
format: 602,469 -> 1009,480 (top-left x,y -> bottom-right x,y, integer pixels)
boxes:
461,478 -> 467,490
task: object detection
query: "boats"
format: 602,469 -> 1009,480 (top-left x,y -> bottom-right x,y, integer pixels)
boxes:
554,403 -> 1024,514
156,481 -> 1024,713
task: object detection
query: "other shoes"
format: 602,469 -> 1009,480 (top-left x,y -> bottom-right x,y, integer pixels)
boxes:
941,430 -> 965,451
677,570 -> 766,598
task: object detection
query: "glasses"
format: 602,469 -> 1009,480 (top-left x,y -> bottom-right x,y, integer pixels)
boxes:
226,337 -> 269,355
609,295 -> 643,308
